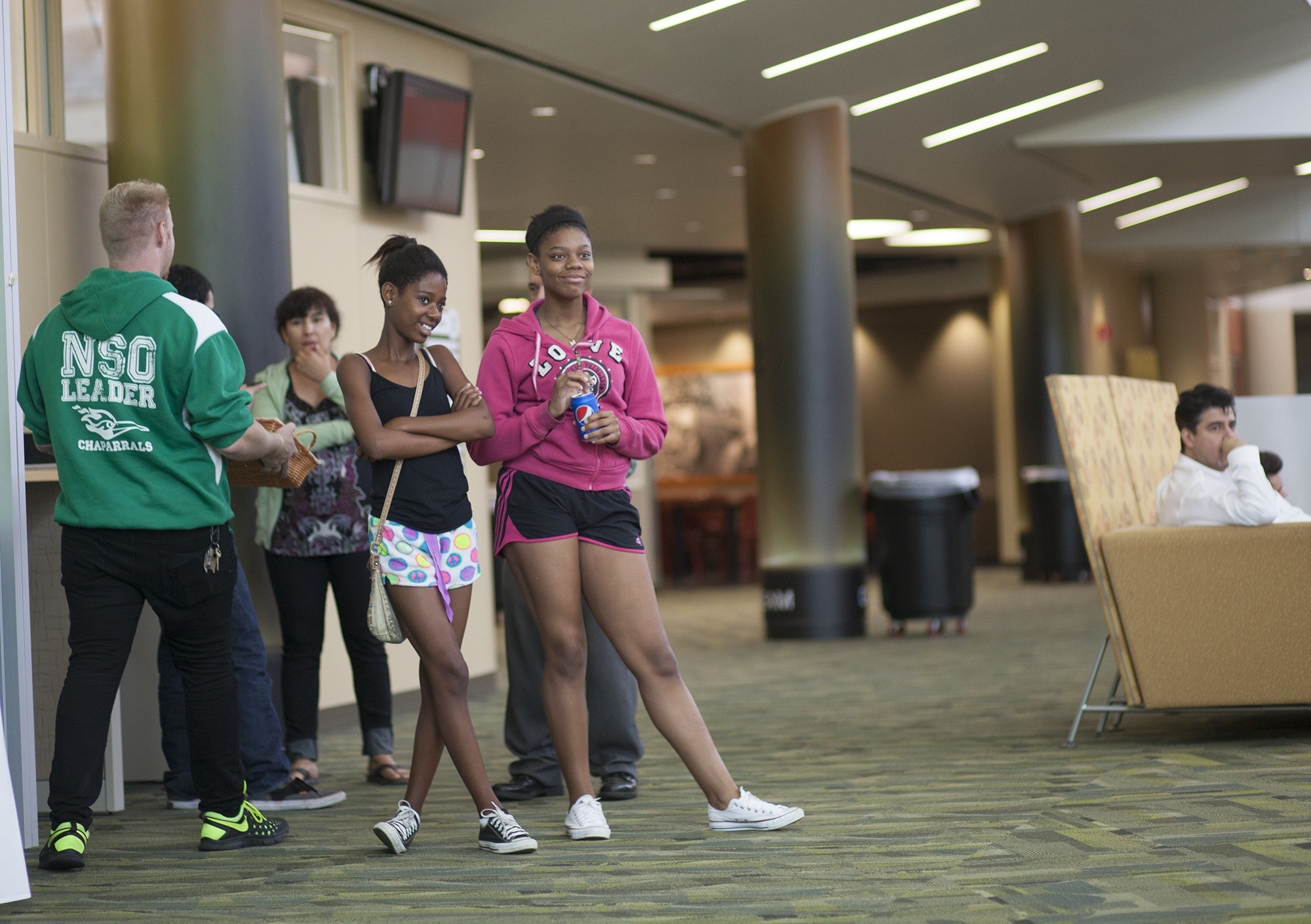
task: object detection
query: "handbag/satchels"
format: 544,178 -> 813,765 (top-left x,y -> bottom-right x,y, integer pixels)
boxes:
366,559 -> 403,645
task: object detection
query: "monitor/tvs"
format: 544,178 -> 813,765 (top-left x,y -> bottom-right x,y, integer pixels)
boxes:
374,73 -> 472,216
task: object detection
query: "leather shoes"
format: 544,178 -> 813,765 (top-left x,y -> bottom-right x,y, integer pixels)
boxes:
489,772 -> 564,799
598,772 -> 638,799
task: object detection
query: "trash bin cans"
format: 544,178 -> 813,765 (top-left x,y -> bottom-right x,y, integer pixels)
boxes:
1023,469 -> 1090,581
865,469 -> 982,637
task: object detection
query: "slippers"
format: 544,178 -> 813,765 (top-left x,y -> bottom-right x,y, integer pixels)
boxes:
271,766 -> 320,788
365,761 -> 412,786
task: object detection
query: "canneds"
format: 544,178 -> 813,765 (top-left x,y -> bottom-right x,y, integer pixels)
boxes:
571,391 -> 604,442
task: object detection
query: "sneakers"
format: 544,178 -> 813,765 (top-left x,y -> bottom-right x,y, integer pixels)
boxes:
478,801 -> 538,854
374,799 -> 422,852
249,781 -> 346,810
706,786 -> 805,833
196,801 -> 291,851
168,794 -> 202,809
564,795 -> 613,838
35,819 -> 90,865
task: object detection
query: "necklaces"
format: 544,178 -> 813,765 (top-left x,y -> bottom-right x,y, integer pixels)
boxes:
539,306 -> 586,348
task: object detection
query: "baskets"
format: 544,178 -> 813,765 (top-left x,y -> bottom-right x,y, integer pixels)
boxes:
228,419 -> 322,488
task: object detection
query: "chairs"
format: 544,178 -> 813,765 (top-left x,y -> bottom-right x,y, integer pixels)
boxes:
1046,374 -> 1311,747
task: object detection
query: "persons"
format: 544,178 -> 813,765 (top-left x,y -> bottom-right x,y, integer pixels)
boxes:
16,180 -> 298,866
1153,383 -> 1311,527
465,205 -> 805,839
156,265 -> 645,809
338,235 -> 538,855
